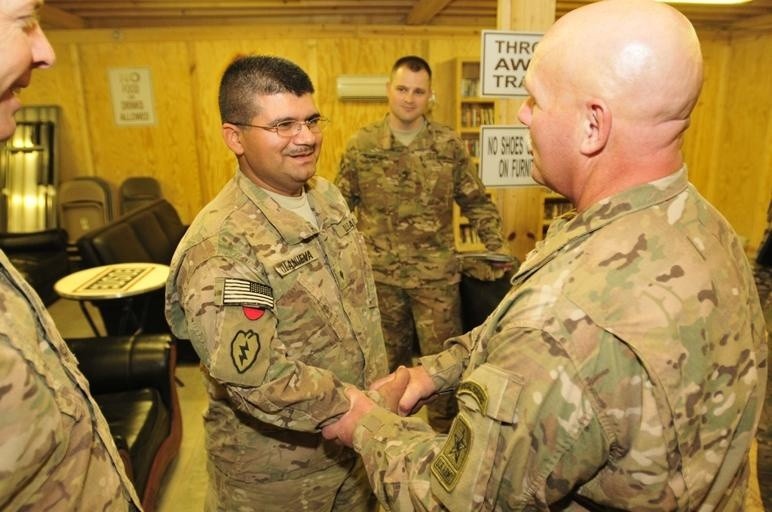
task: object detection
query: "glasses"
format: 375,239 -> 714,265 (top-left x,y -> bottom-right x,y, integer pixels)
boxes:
226,117 -> 331,138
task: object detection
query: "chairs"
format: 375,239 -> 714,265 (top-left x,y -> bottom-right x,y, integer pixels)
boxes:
60,334 -> 184,511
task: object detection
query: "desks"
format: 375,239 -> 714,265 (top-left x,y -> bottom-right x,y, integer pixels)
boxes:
53,263 -> 186,388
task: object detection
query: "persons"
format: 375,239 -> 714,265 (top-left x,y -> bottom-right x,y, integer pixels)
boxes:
165,53 -> 410,511
1,0 -> 149,512
320,0 -> 769,512
330,54 -> 511,437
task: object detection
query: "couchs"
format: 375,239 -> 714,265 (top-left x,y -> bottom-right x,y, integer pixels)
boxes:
76,197 -> 201,366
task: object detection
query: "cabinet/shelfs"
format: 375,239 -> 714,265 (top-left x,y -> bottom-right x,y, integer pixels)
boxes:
444,57 -> 494,250
